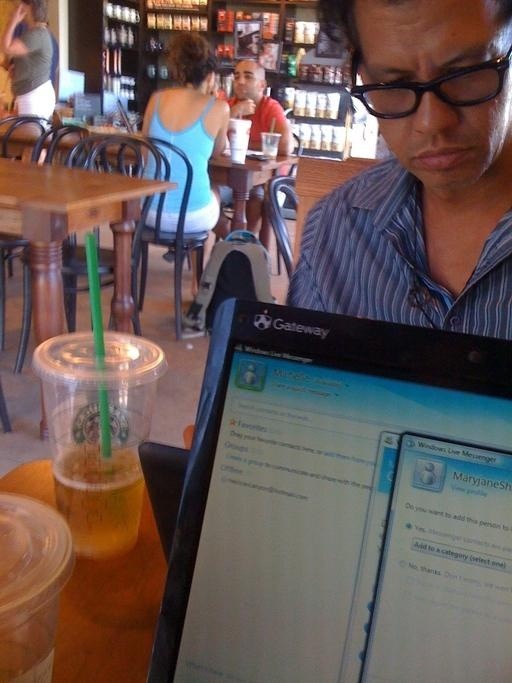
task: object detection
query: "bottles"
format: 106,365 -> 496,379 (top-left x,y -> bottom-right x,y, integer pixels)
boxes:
287,52 -> 297,77
104,2 -> 140,101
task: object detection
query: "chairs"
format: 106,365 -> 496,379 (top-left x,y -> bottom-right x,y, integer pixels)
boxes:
2,113 -> 303,374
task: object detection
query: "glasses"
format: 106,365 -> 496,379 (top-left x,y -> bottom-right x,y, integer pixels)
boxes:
351,47 -> 511,120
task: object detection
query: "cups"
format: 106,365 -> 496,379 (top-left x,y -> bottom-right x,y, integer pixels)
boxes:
216,74 -> 233,97
229,118 -> 252,163
31,326 -> 168,563
159,64 -> 169,80
261,131 -> 282,160
145,64 -> 158,80
283,17 -> 295,41
0,489 -> 79,682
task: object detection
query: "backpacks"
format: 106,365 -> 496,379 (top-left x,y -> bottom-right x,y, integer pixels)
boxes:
193,231 -> 279,339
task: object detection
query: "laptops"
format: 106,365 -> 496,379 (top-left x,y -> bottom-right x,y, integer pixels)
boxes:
146,298 -> 512,683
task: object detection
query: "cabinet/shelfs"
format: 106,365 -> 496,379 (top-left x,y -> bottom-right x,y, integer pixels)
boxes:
207,1 -> 354,163
142,0 -> 208,83
65,0 -> 140,101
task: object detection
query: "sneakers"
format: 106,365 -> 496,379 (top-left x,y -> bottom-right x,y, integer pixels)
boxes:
180,311 -> 209,338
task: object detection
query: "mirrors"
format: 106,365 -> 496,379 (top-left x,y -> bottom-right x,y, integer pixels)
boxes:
233,19 -> 264,61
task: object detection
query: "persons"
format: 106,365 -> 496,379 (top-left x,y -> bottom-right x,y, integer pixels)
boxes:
285,1 -> 512,344
1,1 -> 56,164
140,31 -> 230,298
212,58 -> 295,254
11,17 -> 59,84
